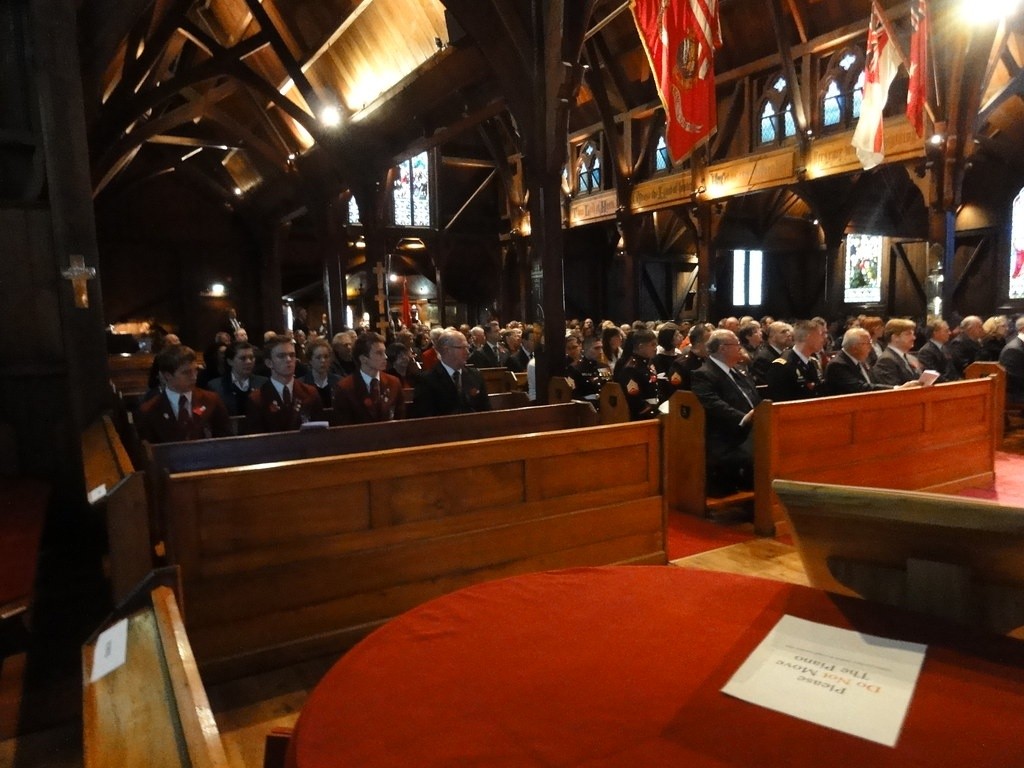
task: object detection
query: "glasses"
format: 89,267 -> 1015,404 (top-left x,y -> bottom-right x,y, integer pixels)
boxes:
448,345 -> 470,351
723,341 -> 742,349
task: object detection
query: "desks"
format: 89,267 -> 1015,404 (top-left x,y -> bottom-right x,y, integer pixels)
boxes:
283,566 -> 1024,768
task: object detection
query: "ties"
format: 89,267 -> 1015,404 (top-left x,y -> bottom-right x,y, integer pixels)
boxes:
492,345 -> 498,361
730,368 -> 758,408
529,353 -> 533,359
370,377 -> 380,410
178,395 -> 193,433
282,385 -> 293,406
452,370 -> 461,391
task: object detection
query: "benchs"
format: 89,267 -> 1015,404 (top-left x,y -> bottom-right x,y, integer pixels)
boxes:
661,389 -> 754,521
127,388 -> 532,470
600,382 -> 630,425
139,399 -> 599,568
547,377 -> 571,404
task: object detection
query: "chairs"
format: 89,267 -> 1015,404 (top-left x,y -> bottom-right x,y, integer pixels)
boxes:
771,477 -> 1024,640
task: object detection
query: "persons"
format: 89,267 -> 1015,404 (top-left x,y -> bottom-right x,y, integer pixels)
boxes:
135,307 -> 1024,498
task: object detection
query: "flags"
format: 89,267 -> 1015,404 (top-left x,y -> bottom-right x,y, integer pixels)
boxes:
851,0 -> 904,171
905,0 -> 932,139
402,279 -> 413,330
629,0 -> 723,170
